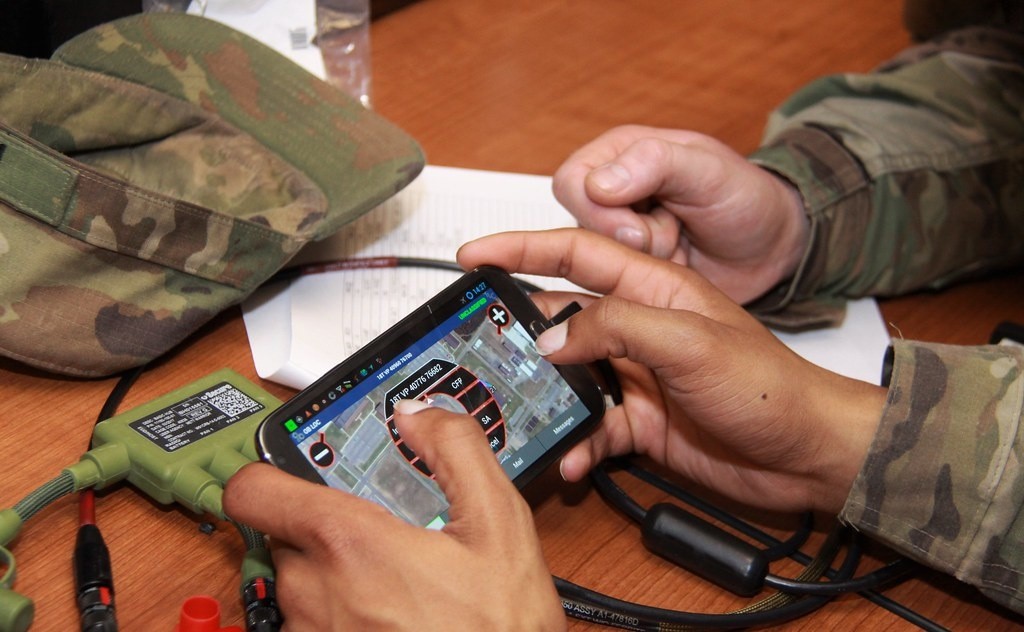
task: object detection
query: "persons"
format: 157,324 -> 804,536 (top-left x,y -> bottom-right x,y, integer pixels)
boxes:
222,228 -> 1024,632
550,0 -> 1024,332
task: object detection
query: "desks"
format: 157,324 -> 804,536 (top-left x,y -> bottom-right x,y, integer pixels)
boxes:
0,0 -> 1024,632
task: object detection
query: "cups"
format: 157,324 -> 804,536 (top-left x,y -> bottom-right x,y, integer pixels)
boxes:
314,1 -> 373,109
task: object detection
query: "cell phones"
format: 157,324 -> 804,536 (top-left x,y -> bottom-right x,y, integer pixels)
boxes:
255,264 -> 605,531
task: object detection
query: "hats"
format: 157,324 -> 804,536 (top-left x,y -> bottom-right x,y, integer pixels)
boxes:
0,9 -> 427,378
